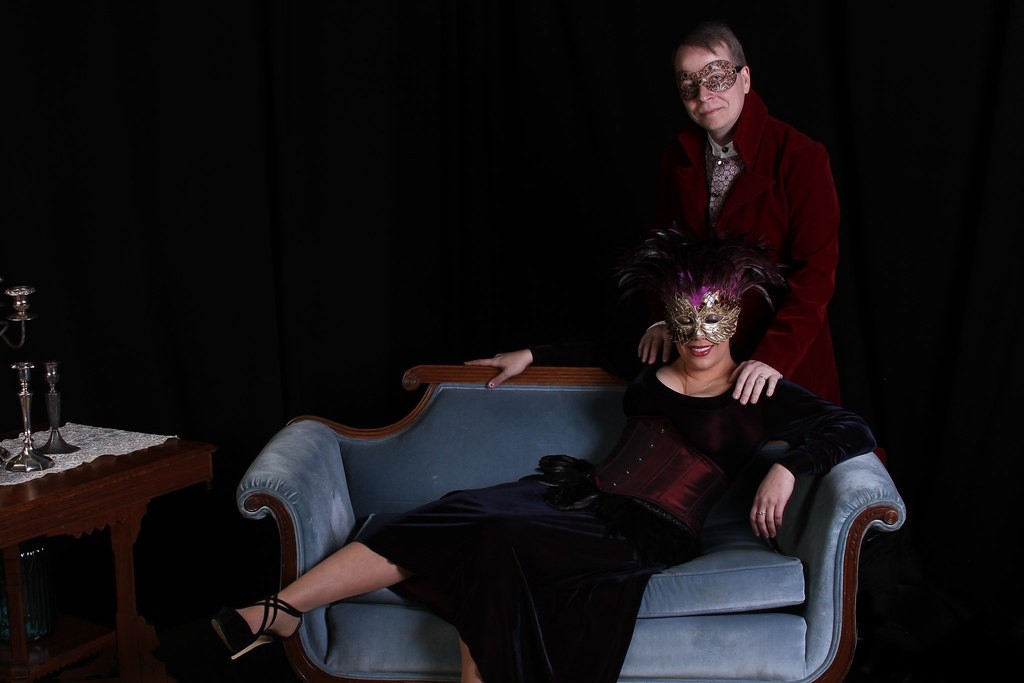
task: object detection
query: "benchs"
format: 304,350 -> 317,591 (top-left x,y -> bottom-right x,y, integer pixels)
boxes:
235,366 -> 907,683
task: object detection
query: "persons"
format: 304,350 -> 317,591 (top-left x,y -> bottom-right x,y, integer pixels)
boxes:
212,226 -> 876,683
638,25 -> 840,406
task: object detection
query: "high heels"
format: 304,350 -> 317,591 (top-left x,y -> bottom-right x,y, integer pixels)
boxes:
212,593 -> 305,661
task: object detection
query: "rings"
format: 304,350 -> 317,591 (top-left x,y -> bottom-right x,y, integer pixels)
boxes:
756,511 -> 765,515
759,376 -> 766,381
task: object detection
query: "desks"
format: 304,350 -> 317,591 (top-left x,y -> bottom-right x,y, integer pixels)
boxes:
0,423 -> 214,680
